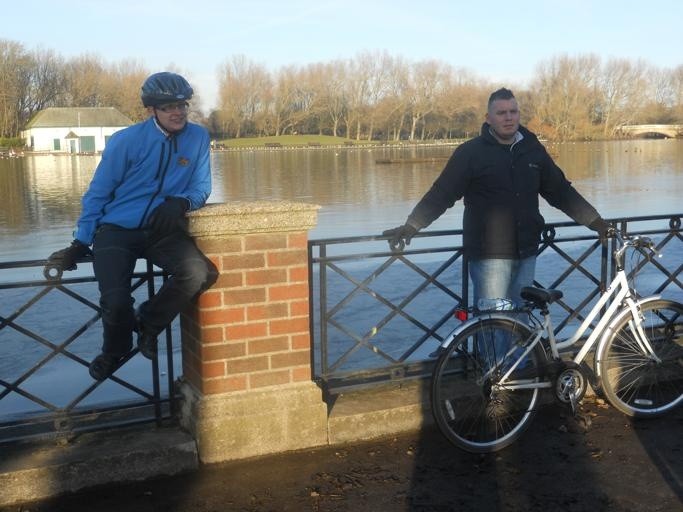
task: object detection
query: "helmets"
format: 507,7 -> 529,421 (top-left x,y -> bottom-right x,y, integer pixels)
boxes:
140,71 -> 196,108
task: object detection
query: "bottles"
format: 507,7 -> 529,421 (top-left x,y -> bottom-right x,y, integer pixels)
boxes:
476,297 -> 517,311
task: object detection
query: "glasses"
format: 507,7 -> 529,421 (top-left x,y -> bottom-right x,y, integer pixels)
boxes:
151,103 -> 192,114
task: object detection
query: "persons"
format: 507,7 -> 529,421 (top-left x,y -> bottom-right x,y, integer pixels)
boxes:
382,86 -> 617,399
49,71 -> 213,381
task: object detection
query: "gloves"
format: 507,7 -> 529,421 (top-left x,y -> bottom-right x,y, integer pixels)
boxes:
145,196 -> 191,235
382,223 -> 419,247
47,239 -> 94,271
589,218 -> 620,242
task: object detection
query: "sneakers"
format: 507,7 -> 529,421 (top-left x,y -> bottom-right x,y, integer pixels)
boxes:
132,303 -> 158,361
88,348 -> 130,377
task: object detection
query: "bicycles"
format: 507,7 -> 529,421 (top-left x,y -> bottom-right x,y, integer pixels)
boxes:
429,229 -> 683,454
